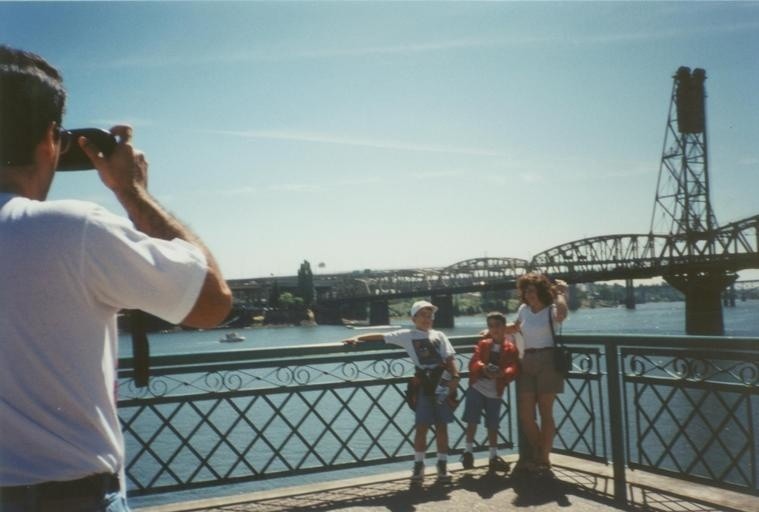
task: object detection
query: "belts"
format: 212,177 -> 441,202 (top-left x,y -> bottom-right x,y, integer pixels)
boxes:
3,476 -> 118,508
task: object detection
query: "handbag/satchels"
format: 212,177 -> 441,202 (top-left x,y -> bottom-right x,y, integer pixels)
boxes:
554,345 -> 572,371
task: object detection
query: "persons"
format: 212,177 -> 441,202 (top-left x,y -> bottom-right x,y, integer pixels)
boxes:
480,272 -> 569,472
462,311 -> 519,470
0,43 -> 233,512
342,301 -> 460,482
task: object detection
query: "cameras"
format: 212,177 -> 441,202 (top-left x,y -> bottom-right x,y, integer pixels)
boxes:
55,127 -> 117,172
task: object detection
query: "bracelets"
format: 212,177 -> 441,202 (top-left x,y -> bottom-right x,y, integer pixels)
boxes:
555,292 -> 566,296
452,375 -> 459,380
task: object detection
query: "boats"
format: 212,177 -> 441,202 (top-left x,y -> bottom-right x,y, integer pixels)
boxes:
220,331 -> 245,343
342,317 -> 370,326
345,326 -> 354,329
300,307 -> 320,328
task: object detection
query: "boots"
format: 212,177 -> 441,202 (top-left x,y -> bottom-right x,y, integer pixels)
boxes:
411,462 -> 425,482
436,462 -> 453,481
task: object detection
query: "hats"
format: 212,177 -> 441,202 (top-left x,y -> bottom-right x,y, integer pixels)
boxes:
412,301 -> 438,316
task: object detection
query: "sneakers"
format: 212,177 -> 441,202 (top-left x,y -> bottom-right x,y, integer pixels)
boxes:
488,457 -> 510,472
459,452 -> 474,468
533,462 -> 551,475
